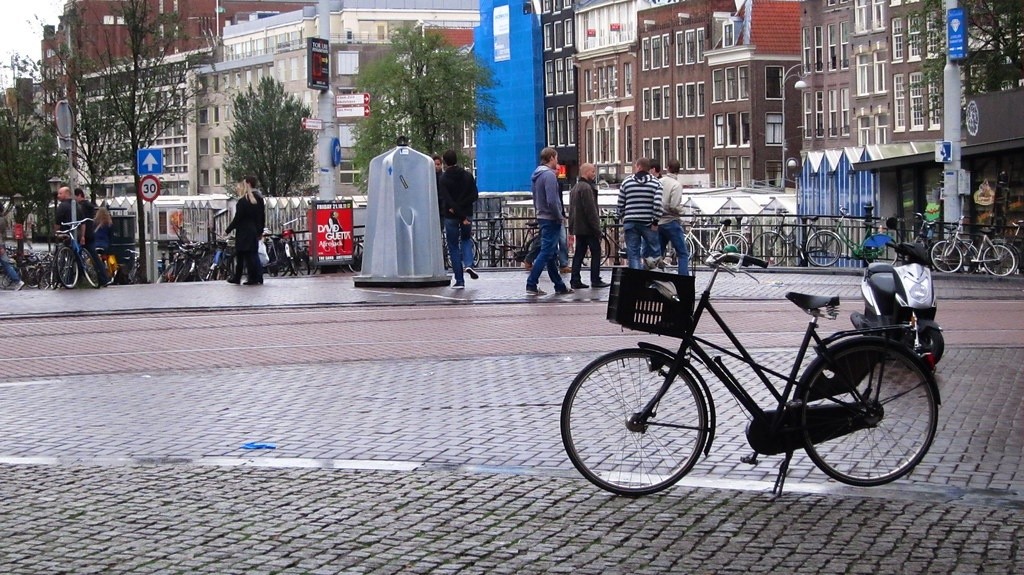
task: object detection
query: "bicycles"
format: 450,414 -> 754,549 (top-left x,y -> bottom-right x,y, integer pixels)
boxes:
1,203 -> 1023,290
560,245 -> 947,498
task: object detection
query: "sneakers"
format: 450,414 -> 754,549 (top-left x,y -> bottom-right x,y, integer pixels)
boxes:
451,281 -> 465,289
555,287 -> 575,294
14,280 -> 25,290
526,288 -> 547,296
465,267 -> 479,279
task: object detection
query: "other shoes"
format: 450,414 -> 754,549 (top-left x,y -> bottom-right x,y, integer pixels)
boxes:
571,283 -> 589,288
244,279 -> 258,285
560,266 -> 572,273
257,278 -> 263,284
590,281 -> 610,287
227,277 -> 240,284
103,276 -> 113,287
50,281 -> 65,289
523,259 -> 533,270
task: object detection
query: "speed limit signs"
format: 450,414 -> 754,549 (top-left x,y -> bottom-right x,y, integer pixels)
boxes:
140,175 -> 160,202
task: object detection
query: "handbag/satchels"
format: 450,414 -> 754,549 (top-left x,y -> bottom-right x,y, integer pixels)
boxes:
257,239 -> 269,267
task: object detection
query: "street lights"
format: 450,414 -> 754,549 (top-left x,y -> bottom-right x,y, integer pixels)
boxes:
593,92 -> 615,189
779,62 -> 809,193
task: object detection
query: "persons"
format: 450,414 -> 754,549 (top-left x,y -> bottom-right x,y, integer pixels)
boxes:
430,154 -> 444,231
73,188 -> 114,288
438,150 -> 479,289
525,163 -> 572,274
246,177 -> 266,285
615,158 -> 664,269
0,202 -> 25,291
53,186 -> 86,288
92,206 -> 113,256
225,179 -> 261,286
525,148 -> 575,296
568,163 -> 611,289
648,159 -> 663,180
657,159 -> 698,293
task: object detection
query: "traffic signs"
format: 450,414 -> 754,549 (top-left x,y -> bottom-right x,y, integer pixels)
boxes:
335,93 -> 371,105
335,106 -> 370,118
301,117 -> 323,130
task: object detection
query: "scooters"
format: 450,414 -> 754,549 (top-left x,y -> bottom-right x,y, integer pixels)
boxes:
849,216 -> 944,371
